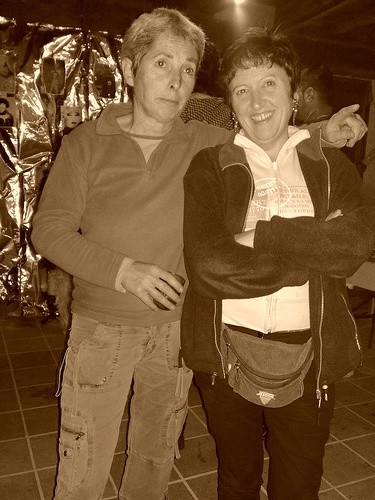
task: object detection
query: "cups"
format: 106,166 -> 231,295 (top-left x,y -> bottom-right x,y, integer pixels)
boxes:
153,271 -> 186,310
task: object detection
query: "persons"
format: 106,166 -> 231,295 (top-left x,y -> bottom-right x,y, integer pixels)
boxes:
31,8 -> 368,500
181,28 -> 375,500
62,107 -> 83,136
177,39 -> 242,131
0,98 -> 14,127
290,59 -> 337,125
